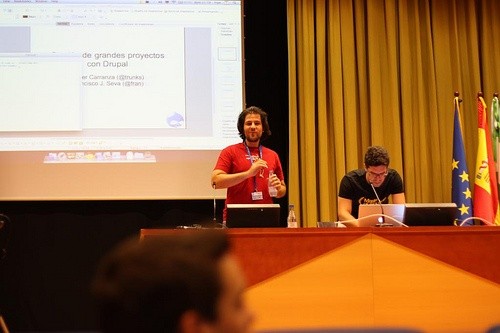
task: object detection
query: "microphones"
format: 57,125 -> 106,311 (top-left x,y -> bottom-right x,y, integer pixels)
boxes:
212,182 -> 216,223
370,182 -> 394,227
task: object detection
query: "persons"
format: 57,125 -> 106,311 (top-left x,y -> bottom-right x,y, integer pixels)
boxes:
338,145 -> 405,226
89,229 -> 256,333
210,106 -> 286,228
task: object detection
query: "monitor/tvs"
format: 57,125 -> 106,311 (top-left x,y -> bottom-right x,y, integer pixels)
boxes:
404,203 -> 458,227
225,204 -> 280,229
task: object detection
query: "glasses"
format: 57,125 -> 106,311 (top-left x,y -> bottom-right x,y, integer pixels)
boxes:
366,166 -> 388,178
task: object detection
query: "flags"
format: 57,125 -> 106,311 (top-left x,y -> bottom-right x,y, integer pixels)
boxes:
451,94 -> 500,226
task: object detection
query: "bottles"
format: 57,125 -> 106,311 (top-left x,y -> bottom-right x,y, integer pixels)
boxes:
287,205 -> 297,229
268,170 -> 278,197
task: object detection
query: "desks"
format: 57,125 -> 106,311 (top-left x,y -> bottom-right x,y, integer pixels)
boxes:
140,226 -> 500,333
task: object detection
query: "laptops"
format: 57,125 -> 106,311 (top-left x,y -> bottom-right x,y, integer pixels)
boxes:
358,204 -> 404,228
